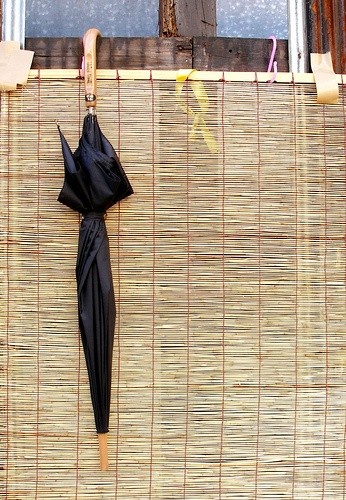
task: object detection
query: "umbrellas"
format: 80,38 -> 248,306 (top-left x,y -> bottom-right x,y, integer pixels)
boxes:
56,28 -> 134,472
